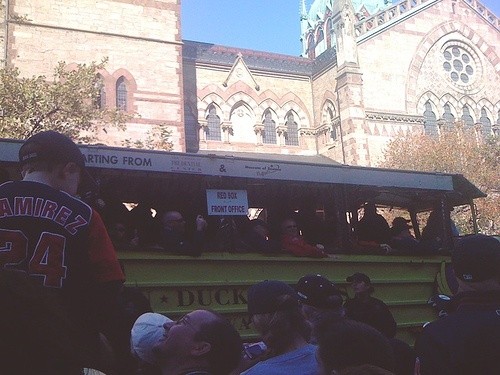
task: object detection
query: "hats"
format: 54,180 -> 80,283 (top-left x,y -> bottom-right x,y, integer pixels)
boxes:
247,279 -> 297,314
393,217 -> 411,227
130,312 -> 175,363
20,129 -> 99,193
346,272 -> 370,283
452,234 -> 500,282
432,199 -> 454,211
295,273 -> 343,307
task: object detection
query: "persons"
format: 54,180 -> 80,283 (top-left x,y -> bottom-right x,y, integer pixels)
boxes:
0,129 -> 132,374
85,274 -> 416,375
77,173 -> 459,257
416,232 -> 500,375
343,272 -> 399,339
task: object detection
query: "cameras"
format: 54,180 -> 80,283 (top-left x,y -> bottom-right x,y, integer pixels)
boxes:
243,341 -> 268,359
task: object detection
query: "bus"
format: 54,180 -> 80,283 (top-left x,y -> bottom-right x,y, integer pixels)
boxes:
0,138 -> 500,351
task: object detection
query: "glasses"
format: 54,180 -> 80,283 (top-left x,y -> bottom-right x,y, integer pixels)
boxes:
179,314 -> 206,340
169,218 -> 185,225
285,224 -> 299,230
